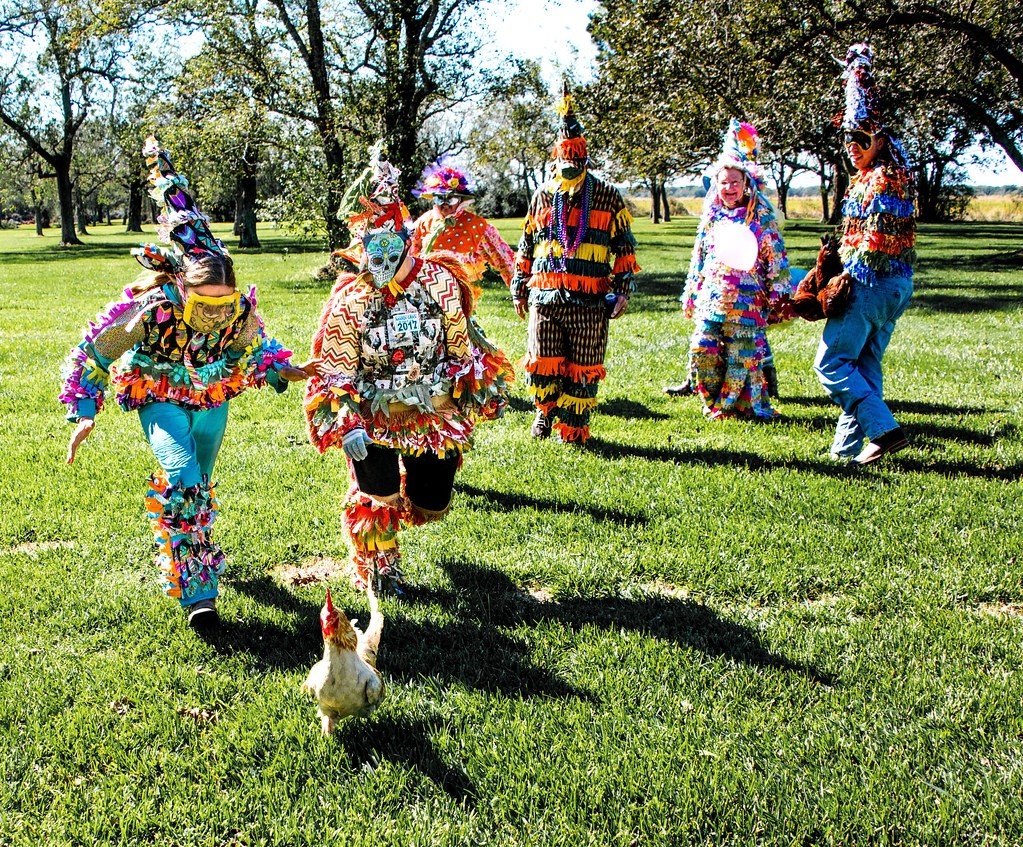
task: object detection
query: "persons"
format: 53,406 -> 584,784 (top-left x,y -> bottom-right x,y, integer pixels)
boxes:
666,148 -> 790,420
408,172 -> 518,420
814,110 -> 916,468
510,129 -> 634,448
61,253 -> 330,629
308,220 -> 475,585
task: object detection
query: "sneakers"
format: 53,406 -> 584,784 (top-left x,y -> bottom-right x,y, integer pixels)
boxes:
532,411 -> 552,439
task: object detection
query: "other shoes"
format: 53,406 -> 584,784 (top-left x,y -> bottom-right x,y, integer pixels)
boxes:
188,599 -> 220,629
848,427 -> 909,466
665,381 -> 692,397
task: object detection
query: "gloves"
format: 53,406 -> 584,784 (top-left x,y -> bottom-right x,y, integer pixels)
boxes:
341,429 -> 373,461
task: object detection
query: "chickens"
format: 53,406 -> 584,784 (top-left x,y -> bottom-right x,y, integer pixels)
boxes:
300,573 -> 386,737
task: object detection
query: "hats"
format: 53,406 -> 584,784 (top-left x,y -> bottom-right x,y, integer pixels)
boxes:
413,156 -> 475,198
705,118 -> 767,189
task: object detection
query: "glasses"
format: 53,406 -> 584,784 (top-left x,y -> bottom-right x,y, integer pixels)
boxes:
432,196 -> 459,206
